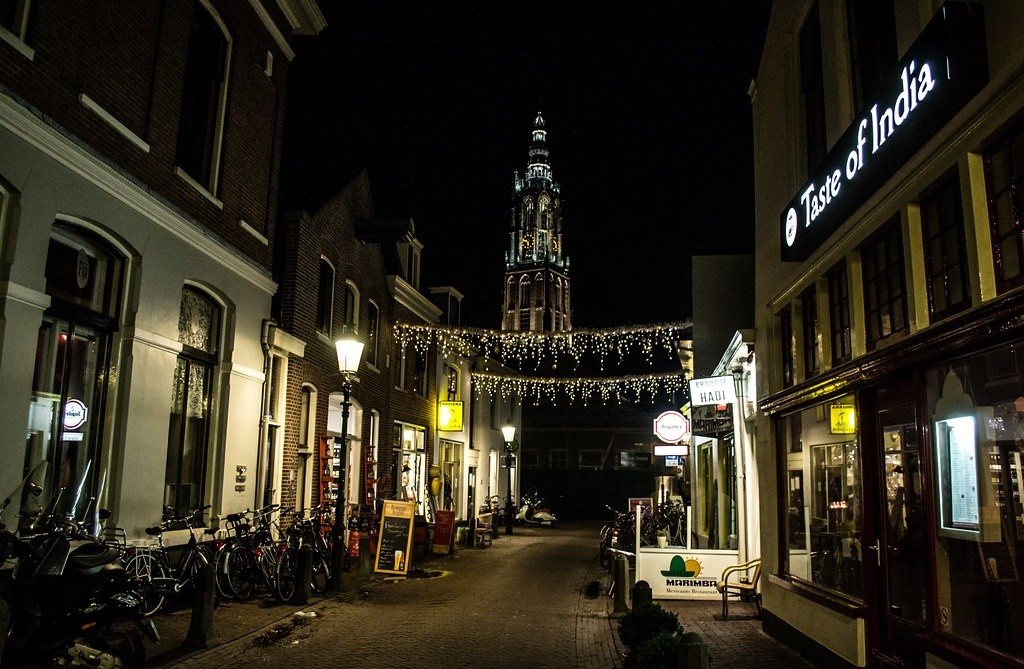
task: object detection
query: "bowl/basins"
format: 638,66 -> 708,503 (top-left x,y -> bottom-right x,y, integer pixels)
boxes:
482,523 -> 492,528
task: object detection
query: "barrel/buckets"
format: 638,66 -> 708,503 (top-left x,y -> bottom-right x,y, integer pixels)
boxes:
457,527 -> 470,542
477,513 -> 493,523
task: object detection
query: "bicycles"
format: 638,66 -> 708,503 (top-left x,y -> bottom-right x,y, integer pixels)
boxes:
480,495 -> 519,526
118,499 -> 351,616
600,497 -> 699,573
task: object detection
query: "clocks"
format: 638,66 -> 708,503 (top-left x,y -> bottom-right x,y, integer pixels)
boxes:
552,238 -> 559,252
521,234 -> 534,249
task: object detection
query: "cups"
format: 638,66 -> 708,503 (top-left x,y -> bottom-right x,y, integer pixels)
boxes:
394,550 -> 402,570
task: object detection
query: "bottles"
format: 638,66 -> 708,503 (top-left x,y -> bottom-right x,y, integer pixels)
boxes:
324,479 -> 338,552
367,452 -> 374,479
990,452 -> 1020,507
367,487 -> 374,498
322,438 -> 340,476
431,477 -> 440,495
429,464 -> 439,478
400,552 -> 404,570
348,504 -> 380,535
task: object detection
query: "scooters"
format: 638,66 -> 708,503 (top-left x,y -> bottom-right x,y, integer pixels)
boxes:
0,481 -> 161,669
514,486 -> 558,528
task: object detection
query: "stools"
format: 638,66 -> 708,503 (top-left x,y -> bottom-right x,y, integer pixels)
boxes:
474,528 -> 493,547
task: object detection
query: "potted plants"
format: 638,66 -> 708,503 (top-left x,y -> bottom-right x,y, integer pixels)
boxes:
655,526 -> 670,549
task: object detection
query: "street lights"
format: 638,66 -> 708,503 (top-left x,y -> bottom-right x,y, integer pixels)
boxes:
499,417 -> 516,533
333,324 -> 364,592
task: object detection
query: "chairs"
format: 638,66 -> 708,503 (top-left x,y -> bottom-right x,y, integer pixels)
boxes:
716,556 -> 762,621
